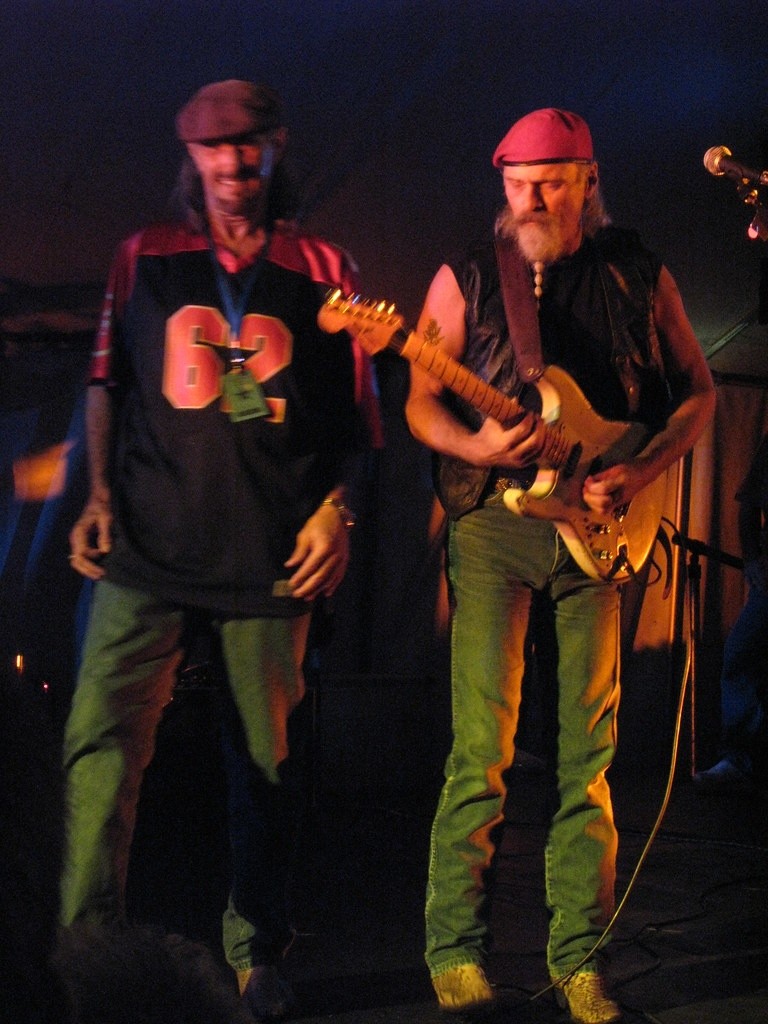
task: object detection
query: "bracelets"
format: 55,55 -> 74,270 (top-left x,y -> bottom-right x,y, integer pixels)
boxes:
322,498 -> 360,533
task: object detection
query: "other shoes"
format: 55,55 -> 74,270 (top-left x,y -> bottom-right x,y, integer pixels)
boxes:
238,966 -> 296,1020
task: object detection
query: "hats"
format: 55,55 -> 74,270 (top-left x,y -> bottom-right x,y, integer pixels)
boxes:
177,79 -> 284,142
492,107 -> 594,170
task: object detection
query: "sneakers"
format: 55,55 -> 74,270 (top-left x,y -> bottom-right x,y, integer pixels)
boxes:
550,971 -> 619,1024
431,964 -> 494,1011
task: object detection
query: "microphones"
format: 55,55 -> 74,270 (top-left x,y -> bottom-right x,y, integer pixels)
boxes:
703,145 -> 768,185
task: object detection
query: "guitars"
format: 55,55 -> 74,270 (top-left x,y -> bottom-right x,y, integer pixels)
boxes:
316,287 -> 668,586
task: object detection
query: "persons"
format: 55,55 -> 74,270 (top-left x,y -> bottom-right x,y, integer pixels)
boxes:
401,110 -> 718,1024
691,431 -> 768,786
50,79 -> 385,1023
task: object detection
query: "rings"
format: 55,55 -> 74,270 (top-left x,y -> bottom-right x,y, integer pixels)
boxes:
611,489 -> 623,503
68,555 -> 76,559
